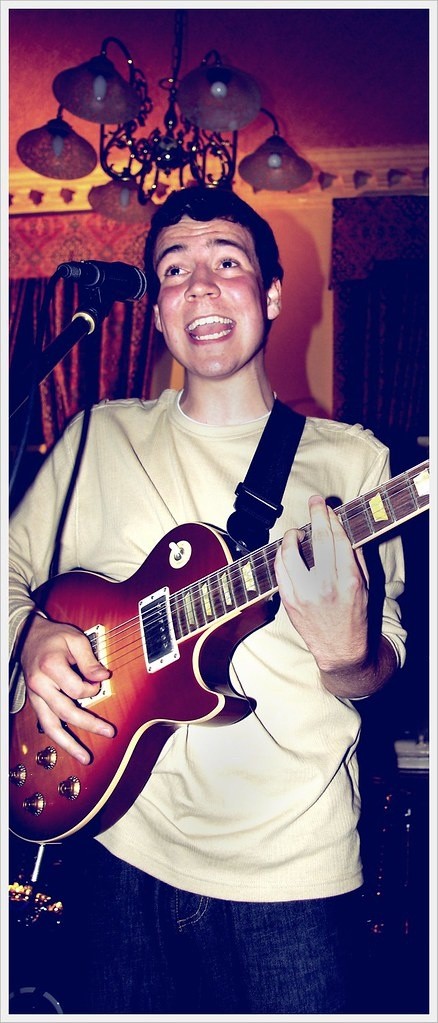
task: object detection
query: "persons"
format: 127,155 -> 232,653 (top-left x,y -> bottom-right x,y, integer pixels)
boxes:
10,185 -> 407,1014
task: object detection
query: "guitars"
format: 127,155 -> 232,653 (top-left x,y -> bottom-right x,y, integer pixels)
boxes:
9,458 -> 429,846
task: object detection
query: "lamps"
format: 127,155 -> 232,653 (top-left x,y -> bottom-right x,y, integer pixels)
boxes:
16,8 -> 317,223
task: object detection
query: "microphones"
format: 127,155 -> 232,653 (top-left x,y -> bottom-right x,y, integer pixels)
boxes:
57,259 -> 149,303
